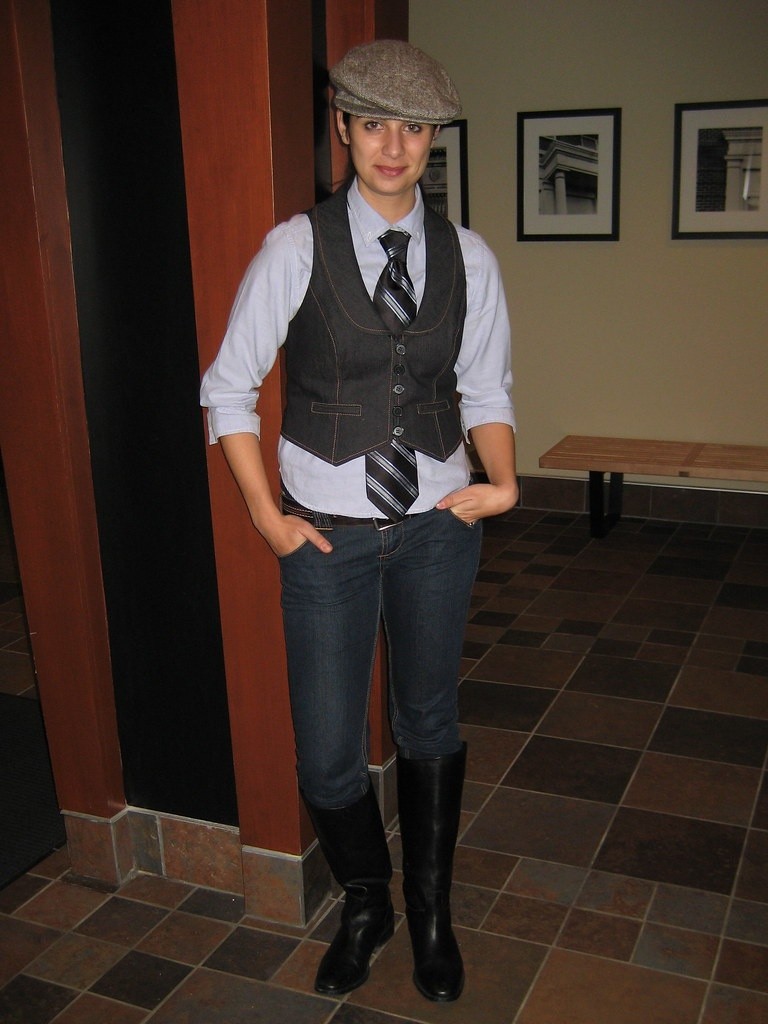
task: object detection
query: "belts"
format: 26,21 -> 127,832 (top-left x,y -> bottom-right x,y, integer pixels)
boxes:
280,492 -> 421,531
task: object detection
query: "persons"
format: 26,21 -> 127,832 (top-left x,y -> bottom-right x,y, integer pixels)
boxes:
199,39 -> 519,1002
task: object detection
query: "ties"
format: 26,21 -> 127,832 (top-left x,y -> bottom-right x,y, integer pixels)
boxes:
366,228 -> 421,523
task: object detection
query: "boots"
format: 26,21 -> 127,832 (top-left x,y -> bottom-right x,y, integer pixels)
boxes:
295,781 -> 396,995
395,736 -> 466,1002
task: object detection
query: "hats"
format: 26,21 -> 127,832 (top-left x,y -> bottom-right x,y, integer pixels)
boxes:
327,39 -> 462,123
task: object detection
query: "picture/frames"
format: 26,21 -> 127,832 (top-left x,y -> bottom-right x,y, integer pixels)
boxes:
515,106 -> 622,242
671,98 -> 768,240
417,119 -> 469,230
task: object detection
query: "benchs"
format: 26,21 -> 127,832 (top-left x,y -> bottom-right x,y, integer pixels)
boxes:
539,435 -> 768,539
